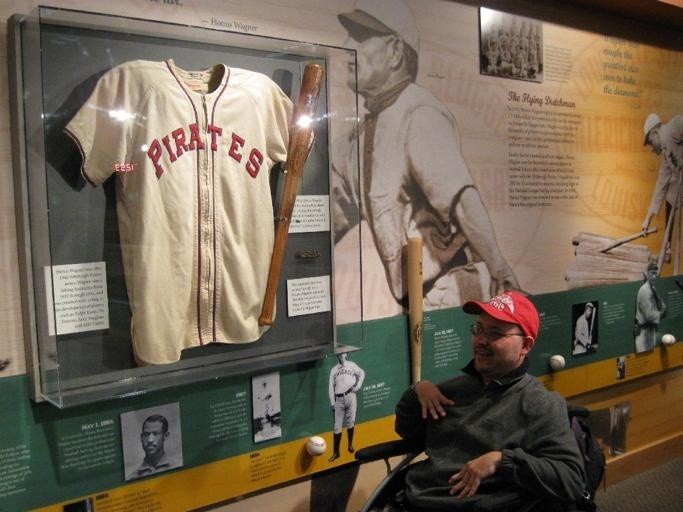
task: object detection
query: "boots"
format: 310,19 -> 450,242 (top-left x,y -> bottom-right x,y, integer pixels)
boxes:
652,241 -> 670,264
347,426 -> 354,453
328,432 -> 342,462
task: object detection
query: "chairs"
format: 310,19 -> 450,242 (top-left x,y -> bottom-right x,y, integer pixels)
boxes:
354,404 -> 606,511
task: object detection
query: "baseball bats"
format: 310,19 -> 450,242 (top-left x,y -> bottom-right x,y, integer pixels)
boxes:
565,229 -> 657,288
260,64 -> 325,326
658,183 -> 682,278
408,238 -> 422,383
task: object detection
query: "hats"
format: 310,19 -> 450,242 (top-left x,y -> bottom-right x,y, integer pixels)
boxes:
462,290 -> 539,342
337,0 -> 420,58
643,113 -> 662,145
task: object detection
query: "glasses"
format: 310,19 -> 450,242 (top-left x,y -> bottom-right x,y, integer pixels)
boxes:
470,323 -> 525,340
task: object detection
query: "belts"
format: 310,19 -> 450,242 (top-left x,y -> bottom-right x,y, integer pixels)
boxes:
396,245 -> 485,310
335,385 -> 355,397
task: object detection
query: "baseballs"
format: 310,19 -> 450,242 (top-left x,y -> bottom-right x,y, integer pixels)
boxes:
662,334 -> 676,347
550,355 -> 565,370
307,437 -> 327,457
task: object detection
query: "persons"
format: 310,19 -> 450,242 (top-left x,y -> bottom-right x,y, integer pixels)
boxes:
330,1 -> 521,315
390,290 -> 587,512
639,111 -> 683,263
571,302 -> 599,356
631,262 -> 667,354
327,351 -> 366,463
123,412 -> 182,481
481,12 -> 544,82
254,379 -> 275,424
